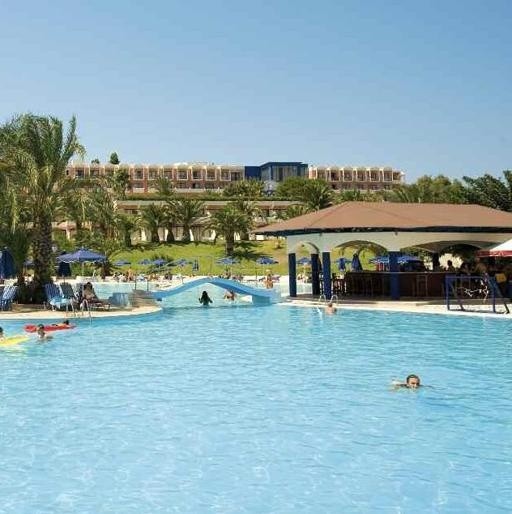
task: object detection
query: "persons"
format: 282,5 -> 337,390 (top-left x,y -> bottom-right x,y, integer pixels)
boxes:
265,274 -> 274,290
223,290 -> 238,300
0,326 -> 25,343
433,257 -> 512,276
199,290 -> 214,306
126,265 -> 134,282
391,373 -> 431,394
83,281 -> 111,311
35,324 -> 54,343
51,319 -> 77,328
325,303 -> 335,313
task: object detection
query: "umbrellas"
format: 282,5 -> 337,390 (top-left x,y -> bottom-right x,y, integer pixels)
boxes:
473,237 -> 511,258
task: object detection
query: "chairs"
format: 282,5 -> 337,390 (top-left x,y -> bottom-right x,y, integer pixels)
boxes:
0,285 -> 19,311
412,274 -> 428,297
440,272 -> 470,297
44,282 -> 111,312
